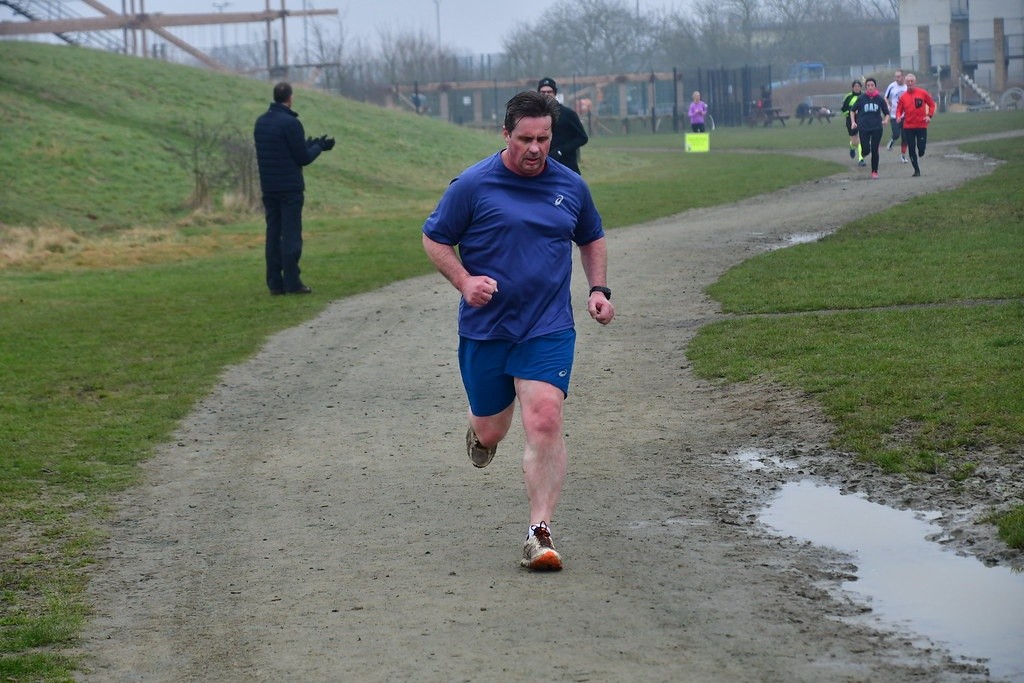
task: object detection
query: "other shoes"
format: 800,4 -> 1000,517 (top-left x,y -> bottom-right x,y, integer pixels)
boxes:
270,289 -> 284,295
284,285 -> 311,293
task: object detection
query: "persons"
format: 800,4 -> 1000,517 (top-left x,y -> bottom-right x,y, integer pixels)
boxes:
850,78 -> 890,178
687,90 -> 709,132
841,80 -> 868,166
896,74 -> 935,177
883,70 -> 909,163
535,78 -> 588,177
253,81 -> 336,294
761,86 -> 773,110
419,91 -> 616,574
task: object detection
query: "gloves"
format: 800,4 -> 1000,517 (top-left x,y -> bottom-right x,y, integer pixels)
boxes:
307,135 -> 319,146
319,134 -> 336,152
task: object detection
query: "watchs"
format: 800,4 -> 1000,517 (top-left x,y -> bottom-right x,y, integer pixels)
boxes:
589,285 -> 611,300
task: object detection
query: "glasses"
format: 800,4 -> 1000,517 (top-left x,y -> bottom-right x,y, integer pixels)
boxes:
540,90 -> 554,94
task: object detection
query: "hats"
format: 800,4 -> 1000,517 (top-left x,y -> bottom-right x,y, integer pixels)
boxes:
537,77 -> 558,95
852,80 -> 862,88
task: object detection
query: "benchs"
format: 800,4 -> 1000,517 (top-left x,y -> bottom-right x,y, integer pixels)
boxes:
796,112 -> 836,124
765,107 -> 790,127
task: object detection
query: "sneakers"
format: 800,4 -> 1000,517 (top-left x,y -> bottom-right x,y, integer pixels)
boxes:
900,154 -> 909,163
919,151 -> 925,156
912,169 -> 921,177
520,526 -> 562,571
465,424 -> 498,470
859,160 -> 867,166
871,171 -> 879,179
887,138 -> 894,151
850,146 -> 856,159
